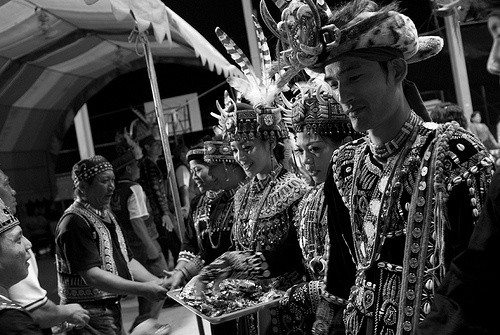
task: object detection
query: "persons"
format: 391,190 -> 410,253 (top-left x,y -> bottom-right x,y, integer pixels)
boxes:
56,155 -> 169,335
430,102 -> 468,131
416,8 -> 500,335
0,169 -> 89,335
0,199 -> 171,335
312,11 -> 493,335
111,152 -> 169,333
470,112 -> 500,156
164,93 -> 362,334
139,134 -> 182,308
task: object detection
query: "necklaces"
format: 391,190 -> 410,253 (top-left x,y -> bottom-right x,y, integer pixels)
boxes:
83,202 -> 108,218
349,143 -> 410,269
236,176 -> 277,251
298,201 -> 329,273
203,198 -> 232,248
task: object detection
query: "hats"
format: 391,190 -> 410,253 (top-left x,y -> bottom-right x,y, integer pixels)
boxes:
72,155 -> 113,186
204,136 -> 235,162
277,81 -> 352,135
186,142 -> 204,162
0,198 -> 20,234
208,91 -> 289,138
260,0 -> 444,87
